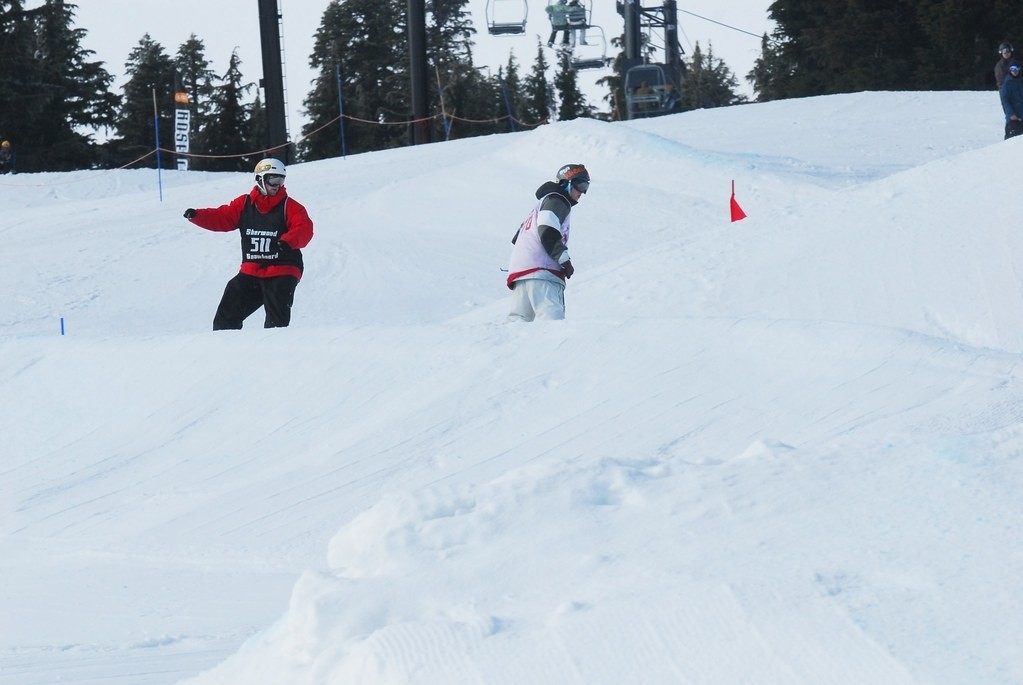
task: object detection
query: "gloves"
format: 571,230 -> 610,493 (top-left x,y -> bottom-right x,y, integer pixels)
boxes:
183,208 -> 196,219
560,260 -> 574,279
271,241 -> 292,256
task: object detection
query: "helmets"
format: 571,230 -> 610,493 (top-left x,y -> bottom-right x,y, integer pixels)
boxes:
556,164 -> 590,182
254,158 -> 286,181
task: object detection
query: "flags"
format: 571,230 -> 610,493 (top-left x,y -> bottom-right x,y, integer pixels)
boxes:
730,195 -> 747,222
174,74 -> 193,171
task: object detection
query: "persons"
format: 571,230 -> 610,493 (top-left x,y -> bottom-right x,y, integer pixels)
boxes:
546,0 -> 587,46
0,141 -> 19,175
506,163 -> 589,323
995,42 -> 1019,87
999,58 -> 1023,139
183,158 -> 315,331
634,80 -> 658,110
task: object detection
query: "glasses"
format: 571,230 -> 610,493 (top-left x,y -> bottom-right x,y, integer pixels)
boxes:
266,175 -> 285,189
571,178 -> 590,194
